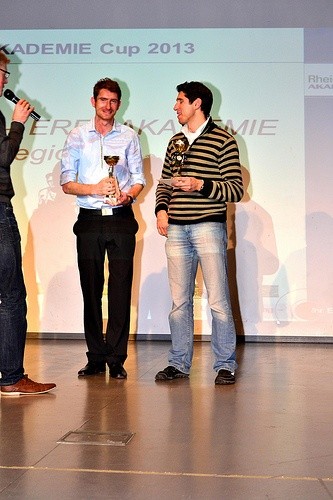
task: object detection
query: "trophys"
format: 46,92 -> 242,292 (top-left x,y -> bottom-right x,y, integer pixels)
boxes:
170,139 -> 189,182
104,155 -> 121,202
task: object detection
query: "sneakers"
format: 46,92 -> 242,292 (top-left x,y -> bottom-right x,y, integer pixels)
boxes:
215,369 -> 235,385
155,365 -> 189,380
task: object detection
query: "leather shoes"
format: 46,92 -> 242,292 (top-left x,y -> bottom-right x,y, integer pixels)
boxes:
109,362 -> 127,379
1,374 -> 57,396
77,360 -> 106,375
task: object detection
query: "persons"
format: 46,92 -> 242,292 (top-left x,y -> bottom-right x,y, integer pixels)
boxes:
60,77 -> 146,380
0,51 -> 57,397
154,81 -> 246,385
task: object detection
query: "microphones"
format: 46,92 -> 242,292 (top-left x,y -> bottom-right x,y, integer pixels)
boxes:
4,89 -> 41,121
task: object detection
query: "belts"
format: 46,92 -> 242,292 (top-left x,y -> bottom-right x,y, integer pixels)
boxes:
79,206 -> 132,216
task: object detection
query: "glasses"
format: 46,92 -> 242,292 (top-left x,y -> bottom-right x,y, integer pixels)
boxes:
0,69 -> 10,78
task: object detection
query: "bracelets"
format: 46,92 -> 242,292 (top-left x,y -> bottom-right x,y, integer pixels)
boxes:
126,193 -> 133,204
